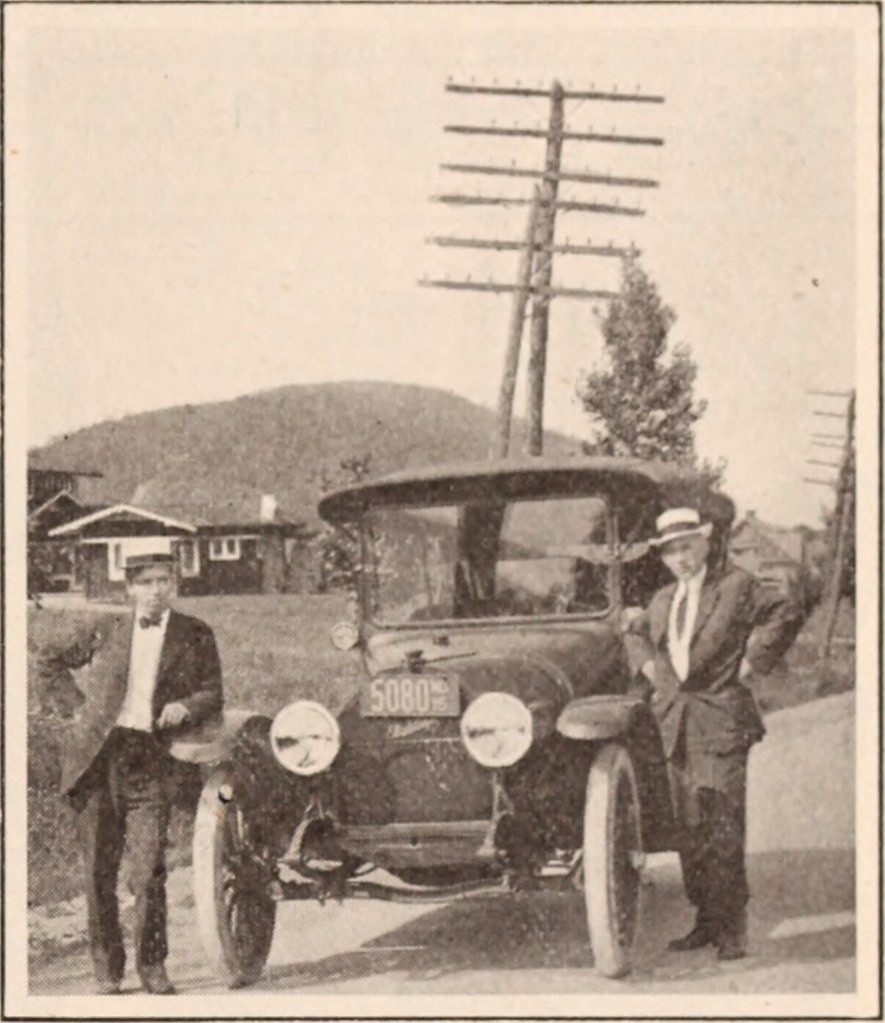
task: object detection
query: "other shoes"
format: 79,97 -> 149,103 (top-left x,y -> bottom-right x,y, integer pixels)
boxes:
97,980 -> 124,995
141,968 -> 173,995
668,928 -> 710,953
715,933 -> 743,960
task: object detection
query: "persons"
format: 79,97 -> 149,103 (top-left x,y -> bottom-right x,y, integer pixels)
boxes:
36,554 -> 224,996
625,507 -> 801,960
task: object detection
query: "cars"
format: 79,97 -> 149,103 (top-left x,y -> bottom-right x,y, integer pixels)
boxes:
170,452 -> 739,989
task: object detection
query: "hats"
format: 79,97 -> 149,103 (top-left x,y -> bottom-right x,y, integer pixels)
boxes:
646,507 -> 713,546
118,536 -> 180,569
